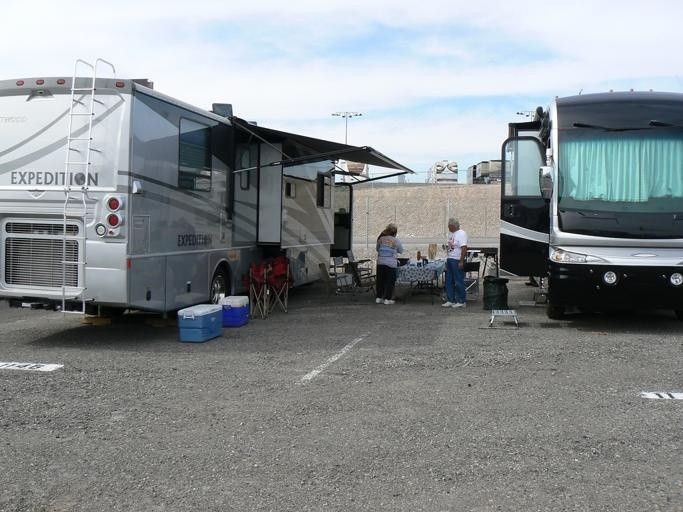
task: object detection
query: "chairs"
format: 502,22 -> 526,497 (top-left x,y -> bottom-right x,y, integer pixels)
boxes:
463,261 -> 481,301
317,250 -> 376,300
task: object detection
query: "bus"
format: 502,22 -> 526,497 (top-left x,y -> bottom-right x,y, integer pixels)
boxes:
0,59 -> 417,327
497,89 -> 683,318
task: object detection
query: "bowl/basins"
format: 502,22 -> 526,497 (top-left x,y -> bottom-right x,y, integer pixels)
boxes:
398,258 -> 409,266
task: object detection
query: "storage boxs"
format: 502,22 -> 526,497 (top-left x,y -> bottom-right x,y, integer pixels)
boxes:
178,295 -> 250,343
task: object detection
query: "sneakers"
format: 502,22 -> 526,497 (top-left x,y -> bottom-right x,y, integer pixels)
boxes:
441,301 -> 455,308
451,302 -> 466,308
384,299 -> 395,305
376,297 -> 384,304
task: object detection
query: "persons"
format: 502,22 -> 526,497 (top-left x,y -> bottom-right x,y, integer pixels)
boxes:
442,217 -> 467,309
374,222 -> 403,305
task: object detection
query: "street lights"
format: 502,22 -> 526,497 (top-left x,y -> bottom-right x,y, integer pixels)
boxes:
331,111 -> 361,145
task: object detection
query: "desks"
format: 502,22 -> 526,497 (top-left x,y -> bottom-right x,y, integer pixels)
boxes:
401,261 -> 442,306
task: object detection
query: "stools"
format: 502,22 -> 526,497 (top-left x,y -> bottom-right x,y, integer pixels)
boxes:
489,310 -> 519,325
